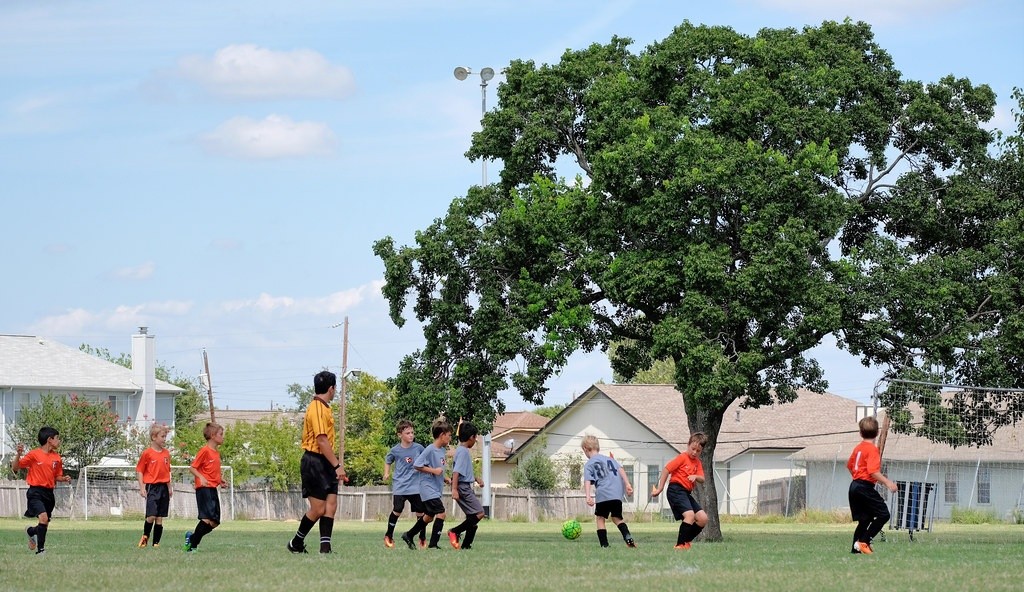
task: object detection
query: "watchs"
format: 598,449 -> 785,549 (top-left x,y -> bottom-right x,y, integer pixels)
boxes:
334,463 -> 341,470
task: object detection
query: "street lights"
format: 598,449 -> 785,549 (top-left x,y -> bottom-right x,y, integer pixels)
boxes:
453,66 -> 490,520
335,369 -> 363,521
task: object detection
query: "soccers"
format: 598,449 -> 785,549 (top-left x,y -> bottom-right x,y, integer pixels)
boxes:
562,519 -> 581,540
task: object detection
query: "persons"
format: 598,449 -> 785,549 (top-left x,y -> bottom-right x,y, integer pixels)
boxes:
401,420 -> 451,550
135,424 -> 172,547
651,432 -> 709,549
11,427 -> 72,555
383,421 -> 452,548
287,371 -> 346,554
846,416 -> 898,554
447,421 -> 485,550
184,423 -> 228,552
580,435 -> 637,549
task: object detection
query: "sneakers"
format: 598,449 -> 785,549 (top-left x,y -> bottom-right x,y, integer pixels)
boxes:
673,542 -> 692,550
853,540 -> 874,554
418,535 -> 428,550
384,535 -> 395,549
447,529 -> 462,550
427,543 -> 442,552
184,531 -> 192,551
25,527 -> 36,550
401,532 -> 417,551
286,539 -> 309,555
139,534 -> 148,549
